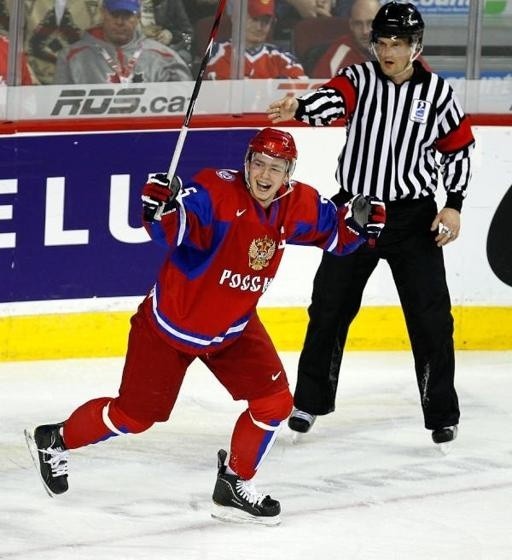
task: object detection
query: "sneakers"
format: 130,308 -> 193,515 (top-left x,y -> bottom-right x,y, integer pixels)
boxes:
432,425 -> 458,444
288,407 -> 317,433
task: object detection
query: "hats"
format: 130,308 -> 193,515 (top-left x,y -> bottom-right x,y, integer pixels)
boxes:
247,1 -> 275,17
104,0 -> 140,15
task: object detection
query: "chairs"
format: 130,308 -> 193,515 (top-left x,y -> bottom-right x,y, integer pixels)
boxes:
192,14 -> 354,82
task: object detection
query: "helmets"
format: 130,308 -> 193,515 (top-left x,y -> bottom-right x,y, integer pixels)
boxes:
369,1 -> 425,57
243,128 -> 298,190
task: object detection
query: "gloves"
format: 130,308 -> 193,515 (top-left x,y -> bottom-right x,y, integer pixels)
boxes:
343,193 -> 388,249
141,170 -> 184,219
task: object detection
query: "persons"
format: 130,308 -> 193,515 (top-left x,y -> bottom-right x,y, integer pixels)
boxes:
34,125 -> 386,516
0,0 -> 434,83
268,0 -> 475,444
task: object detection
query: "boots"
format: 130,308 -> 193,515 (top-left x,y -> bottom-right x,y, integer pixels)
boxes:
211,448 -> 280,517
34,422 -> 70,495
